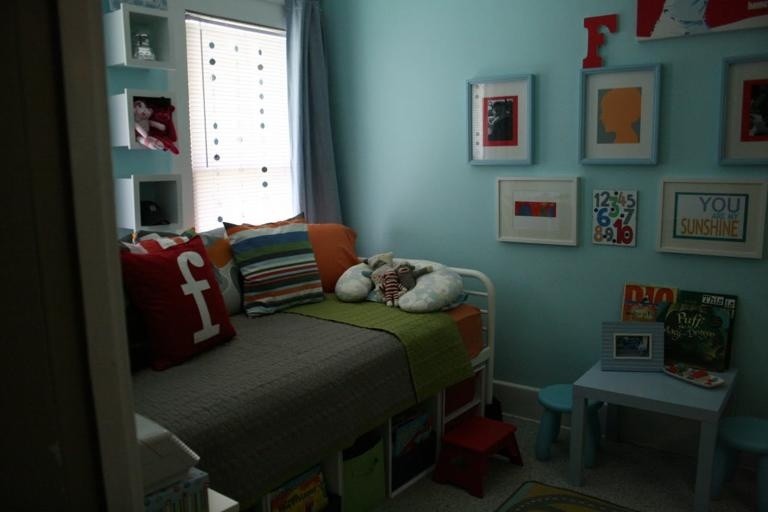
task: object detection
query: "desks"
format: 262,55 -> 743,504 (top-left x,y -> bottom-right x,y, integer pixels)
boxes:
205,487 -> 241,511
568,356 -> 740,511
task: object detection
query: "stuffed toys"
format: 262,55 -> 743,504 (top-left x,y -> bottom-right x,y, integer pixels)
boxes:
363,252 -> 393,286
394,262 -> 433,291
132,99 -> 165,151
131,32 -> 158,62
149,98 -> 179,155
374,270 -> 407,308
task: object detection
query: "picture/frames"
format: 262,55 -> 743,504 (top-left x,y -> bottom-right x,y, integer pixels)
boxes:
599,320 -> 666,374
576,62 -> 665,168
654,176 -> 768,262
716,52 -> 768,167
465,73 -> 537,168
495,176 -> 583,249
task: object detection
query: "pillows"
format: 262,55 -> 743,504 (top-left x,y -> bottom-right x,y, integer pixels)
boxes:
335,256 -> 465,314
118,211 -> 361,375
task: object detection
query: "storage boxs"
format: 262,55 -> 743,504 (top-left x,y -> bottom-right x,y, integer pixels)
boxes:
340,435 -> 389,512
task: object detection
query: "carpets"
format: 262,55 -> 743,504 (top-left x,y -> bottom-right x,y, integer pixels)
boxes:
492,480 -> 640,511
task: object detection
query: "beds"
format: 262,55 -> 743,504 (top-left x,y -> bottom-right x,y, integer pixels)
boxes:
130,255 -> 500,512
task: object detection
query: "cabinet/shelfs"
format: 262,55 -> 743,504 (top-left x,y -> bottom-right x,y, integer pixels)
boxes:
102,3 -> 185,232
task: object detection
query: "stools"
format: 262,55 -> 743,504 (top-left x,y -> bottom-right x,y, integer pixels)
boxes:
709,412 -> 767,511
431,417 -> 525,500
533,383 -> 606,469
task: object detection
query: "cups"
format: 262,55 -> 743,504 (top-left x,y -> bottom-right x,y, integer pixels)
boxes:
133,33 -> 152,59
